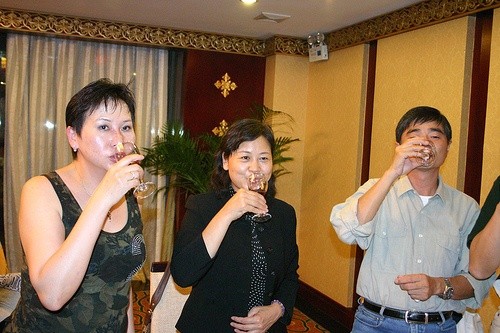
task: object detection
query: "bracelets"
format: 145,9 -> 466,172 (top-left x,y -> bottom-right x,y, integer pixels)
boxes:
271,300 -> 285,317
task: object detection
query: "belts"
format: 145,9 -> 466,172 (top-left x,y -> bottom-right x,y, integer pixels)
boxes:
362,300 -> 451,324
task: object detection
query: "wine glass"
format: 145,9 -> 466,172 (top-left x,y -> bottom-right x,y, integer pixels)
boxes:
114,141 -> 156,200
248,174 -> 272,222
412,141 -> 436,169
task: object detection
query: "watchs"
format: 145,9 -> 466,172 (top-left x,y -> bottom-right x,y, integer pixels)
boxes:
438,277 -> 454,300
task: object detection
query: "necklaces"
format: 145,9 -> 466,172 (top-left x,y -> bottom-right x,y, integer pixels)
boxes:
72,160 -> 112,219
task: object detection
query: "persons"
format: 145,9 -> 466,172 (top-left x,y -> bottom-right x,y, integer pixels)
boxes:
329,106 -> 496,333
467,176 -> 500,333
0,78 -> 148,333
170,118 -> 300,333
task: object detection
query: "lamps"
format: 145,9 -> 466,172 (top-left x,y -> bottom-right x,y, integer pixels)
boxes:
307,32 -> 330,63
